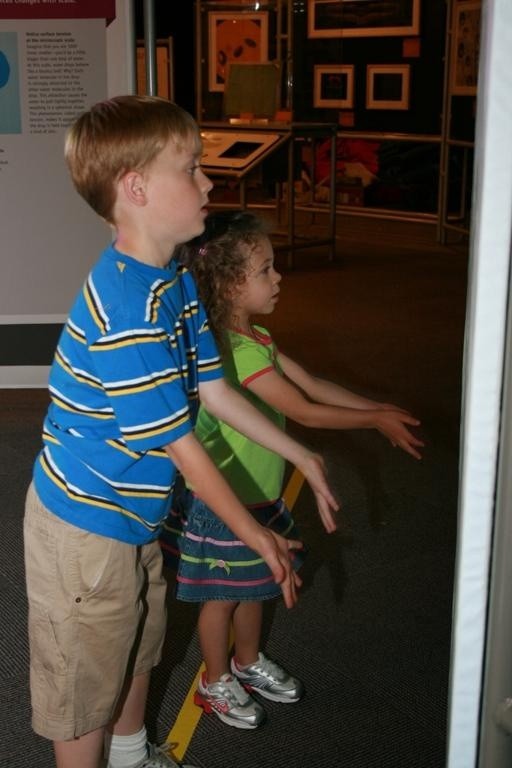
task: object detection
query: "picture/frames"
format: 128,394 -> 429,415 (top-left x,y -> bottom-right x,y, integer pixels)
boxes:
134,37 -> 174,102
307,0 -> 419,40
208,11 -> 268,93
365,64 -> 409,111
448,0 -> 481,96
314,64 -> 354,110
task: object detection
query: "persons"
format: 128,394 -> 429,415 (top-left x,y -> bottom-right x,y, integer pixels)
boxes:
21,93 -> 344,768
158,216 -> 427,732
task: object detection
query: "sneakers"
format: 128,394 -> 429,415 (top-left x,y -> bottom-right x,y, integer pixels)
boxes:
230,652 -> 303,703
198,671 -> 265,730
105,740 -> 180,768
